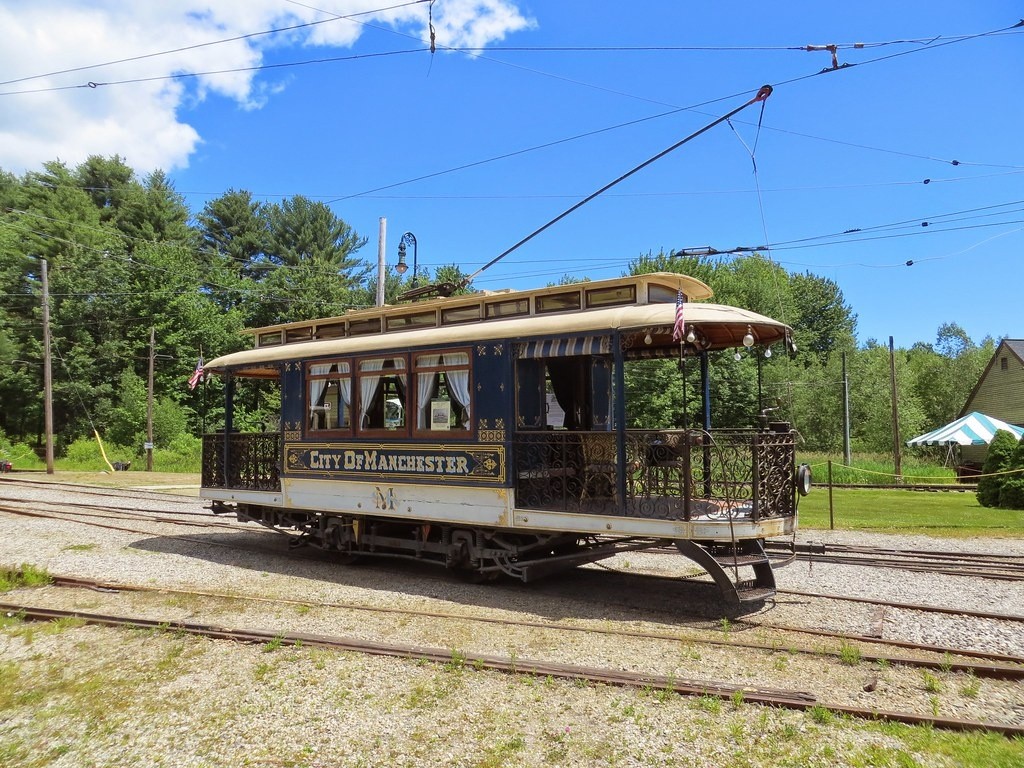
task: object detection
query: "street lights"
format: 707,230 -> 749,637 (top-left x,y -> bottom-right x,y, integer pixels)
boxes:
390,225 -> 420,303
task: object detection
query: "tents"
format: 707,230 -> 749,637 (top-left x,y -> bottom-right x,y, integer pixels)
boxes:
905,410 -> 1023,479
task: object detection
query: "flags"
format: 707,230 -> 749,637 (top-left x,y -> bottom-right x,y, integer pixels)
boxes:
672,287 -> 685,341
187,359 -> 205,390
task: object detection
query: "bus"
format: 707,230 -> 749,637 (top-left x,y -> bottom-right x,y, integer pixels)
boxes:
191,217 -> 816,609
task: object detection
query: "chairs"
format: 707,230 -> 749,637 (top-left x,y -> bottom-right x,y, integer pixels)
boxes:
579,433 -> 639,507
642,428 -> 684,501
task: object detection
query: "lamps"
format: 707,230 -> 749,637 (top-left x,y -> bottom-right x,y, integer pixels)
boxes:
642,325 -> 792,364
